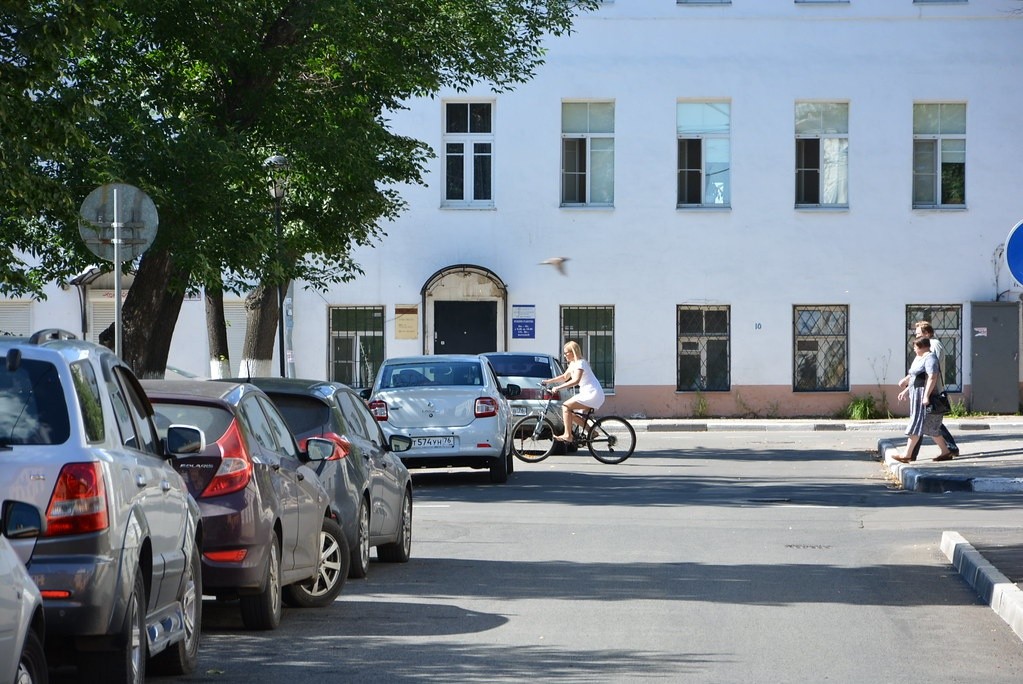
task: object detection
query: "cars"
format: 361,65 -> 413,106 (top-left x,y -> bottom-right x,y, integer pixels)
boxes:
359,353 -> 522,484
471,351 -> 580,436
138,376 -> 352,633
202,375 -> 416,580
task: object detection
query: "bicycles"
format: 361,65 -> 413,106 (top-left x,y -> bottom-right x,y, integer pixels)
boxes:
509,381 -> 636,465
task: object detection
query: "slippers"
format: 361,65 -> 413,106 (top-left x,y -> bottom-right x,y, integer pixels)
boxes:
590,432 -> 599,443
556,435 -> 572,443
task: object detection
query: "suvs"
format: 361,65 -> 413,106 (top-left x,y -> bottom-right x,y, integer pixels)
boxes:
1,326 -> 208,684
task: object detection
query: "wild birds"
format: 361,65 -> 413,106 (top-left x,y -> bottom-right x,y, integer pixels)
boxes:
536,257 -> 568,275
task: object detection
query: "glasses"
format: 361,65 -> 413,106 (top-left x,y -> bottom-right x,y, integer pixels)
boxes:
564,350 -> 572,355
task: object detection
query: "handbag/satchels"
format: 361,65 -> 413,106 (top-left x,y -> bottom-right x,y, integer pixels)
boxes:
923,391 -> 951,414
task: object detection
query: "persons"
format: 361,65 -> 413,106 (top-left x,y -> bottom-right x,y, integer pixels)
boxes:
542,341 -> 605,442
899,321 -> 959,461
891,337 -> 953,464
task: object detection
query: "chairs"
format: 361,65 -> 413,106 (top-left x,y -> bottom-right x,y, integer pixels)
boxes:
528,364 -> 552,377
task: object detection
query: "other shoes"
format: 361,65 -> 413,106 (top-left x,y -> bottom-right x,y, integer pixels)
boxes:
948,443 -> 960,454
891,455 -> 912,463
911,455 -> 917,461
932,452 -> 953,461
